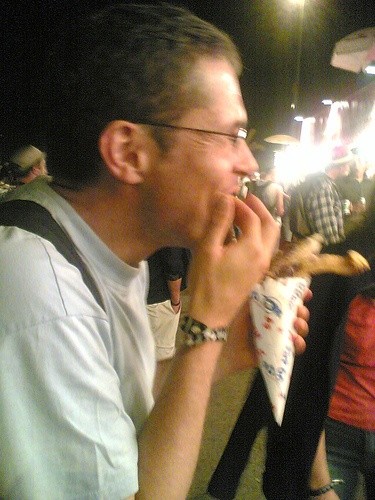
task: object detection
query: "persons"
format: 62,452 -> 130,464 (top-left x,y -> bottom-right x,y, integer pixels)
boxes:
289,144 -> 355,247
0,0 -> 312,500
0,145 -> 51,191
144,245 -> 188,402
203,208 -> 375,500
227,160 -> 285,248
338,148 -> 375,221
324,272 -> 375,500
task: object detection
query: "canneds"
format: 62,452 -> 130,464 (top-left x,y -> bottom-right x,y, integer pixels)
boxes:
359,197 -> 366,205
342,199 -> 350,214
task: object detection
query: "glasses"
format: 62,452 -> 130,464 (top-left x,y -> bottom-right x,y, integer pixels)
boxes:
144,121 -> 247,150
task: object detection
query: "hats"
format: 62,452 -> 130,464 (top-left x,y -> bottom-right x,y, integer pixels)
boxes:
329,147 -> 354,164
11,145 -> 46,180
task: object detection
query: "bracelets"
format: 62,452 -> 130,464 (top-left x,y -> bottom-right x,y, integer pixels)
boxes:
173,315 -> 228,355
171,300 -> 180,306
310,482 -> 335,496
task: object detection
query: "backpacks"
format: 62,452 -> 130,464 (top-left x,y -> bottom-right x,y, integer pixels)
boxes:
251,180 -> 276,214
